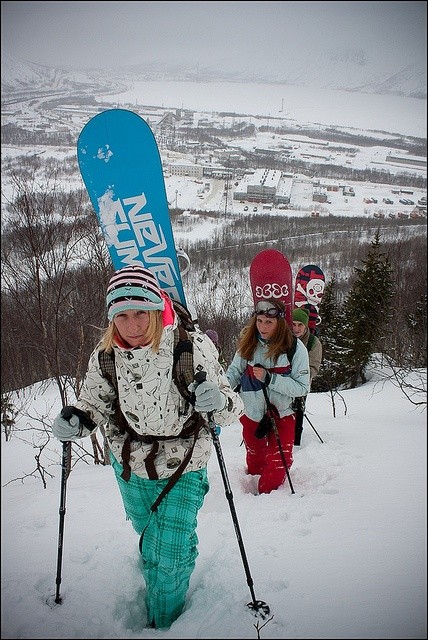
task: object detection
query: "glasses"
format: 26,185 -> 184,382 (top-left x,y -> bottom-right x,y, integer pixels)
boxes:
255,300 -> 278,318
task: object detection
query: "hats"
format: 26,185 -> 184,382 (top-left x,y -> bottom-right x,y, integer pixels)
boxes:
293,308 -> 309,327
106,264 -> 165,320
206,329 -> 218,342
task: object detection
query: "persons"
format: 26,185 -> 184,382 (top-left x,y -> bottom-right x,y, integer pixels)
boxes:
225,299 -> 311,495
205,329 -> 227,376
52,265 -> 246,631
292,306 -> 322,446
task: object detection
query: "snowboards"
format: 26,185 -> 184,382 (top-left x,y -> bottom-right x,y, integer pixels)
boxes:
293,264 -> 326,335
249,248 -> 293,329
74,108 -> 190,308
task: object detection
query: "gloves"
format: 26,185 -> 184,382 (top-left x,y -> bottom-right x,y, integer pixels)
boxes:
188,379 -> 226,412
52,414 -> 81,442
255,405 -> 280,438
